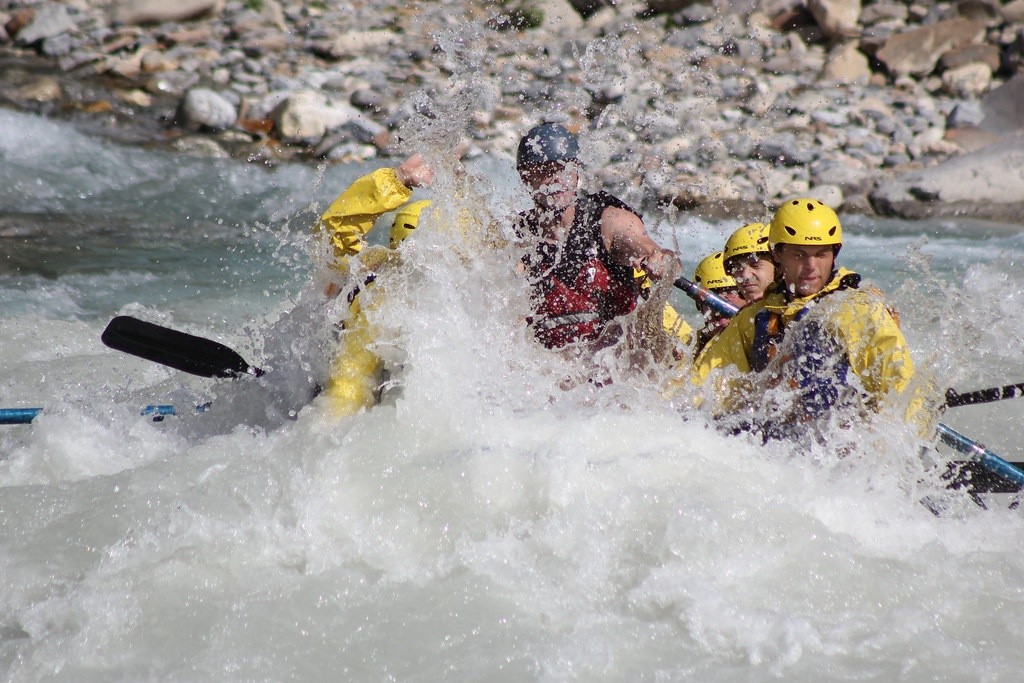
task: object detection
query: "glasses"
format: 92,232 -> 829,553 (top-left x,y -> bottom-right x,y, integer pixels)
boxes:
521,165 -> 568,177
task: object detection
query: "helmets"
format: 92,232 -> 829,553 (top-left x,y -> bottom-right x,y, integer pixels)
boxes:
388,199 -> 433,249
768,197 -> 842,250
722,222 -> 770,276
516,121 -> 580,171
693,251 -> 737,289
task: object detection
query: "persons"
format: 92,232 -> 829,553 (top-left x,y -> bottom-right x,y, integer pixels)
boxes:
661,197 -> 933,443
313,142 -> 485,418
497,122 -> 684,394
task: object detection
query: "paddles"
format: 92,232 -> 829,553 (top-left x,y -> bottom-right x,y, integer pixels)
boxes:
668,276 -> 1024,498
99,313 -> 266,387
